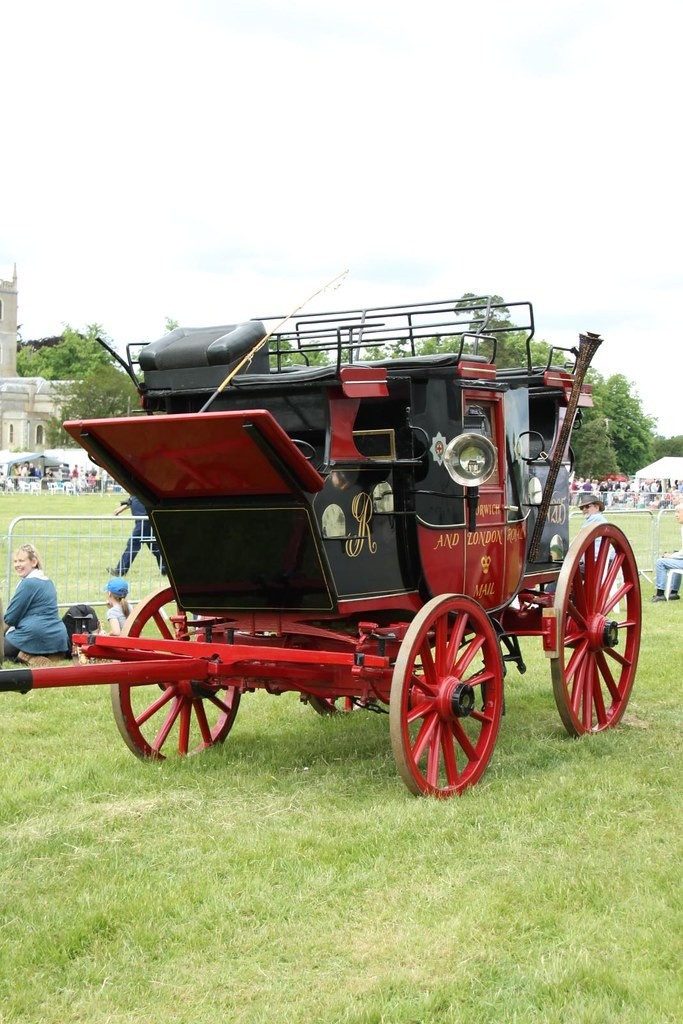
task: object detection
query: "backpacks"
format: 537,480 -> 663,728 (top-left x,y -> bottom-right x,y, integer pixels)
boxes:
63,605 -> 98,659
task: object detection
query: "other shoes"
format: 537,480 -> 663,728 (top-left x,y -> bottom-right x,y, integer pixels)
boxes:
106,568 -> 121,577
161,566 -> 166,576
668,594 -> 680,600
651,595 -> 666,602
14,651 -> 66,667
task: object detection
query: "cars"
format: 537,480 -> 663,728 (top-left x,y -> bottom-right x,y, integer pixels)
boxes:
63,296 -> 642,797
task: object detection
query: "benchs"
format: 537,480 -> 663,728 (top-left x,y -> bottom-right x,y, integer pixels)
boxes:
62,409 -> 325,499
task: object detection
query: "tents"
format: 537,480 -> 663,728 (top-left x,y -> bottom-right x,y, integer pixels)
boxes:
0,448 -> 104,474
635,457 -> 683,498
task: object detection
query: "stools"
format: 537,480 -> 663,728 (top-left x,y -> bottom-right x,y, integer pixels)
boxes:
664,569 -> 683,600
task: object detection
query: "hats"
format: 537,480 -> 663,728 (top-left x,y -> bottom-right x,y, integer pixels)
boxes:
579,495 -> 605,511
102,578 -> 129,595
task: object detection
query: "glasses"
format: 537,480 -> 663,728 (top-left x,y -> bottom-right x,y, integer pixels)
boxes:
580,505 -> 593,510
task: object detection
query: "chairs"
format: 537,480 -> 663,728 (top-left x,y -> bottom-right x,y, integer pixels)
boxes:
0,476 -> 88,496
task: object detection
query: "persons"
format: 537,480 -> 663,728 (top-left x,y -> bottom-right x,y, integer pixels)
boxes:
651,503 -> 683,603
101,577 -> 135,637
570,476 -> 683,509
106,495 -> 169,577
0,462 -> 110,493
3,544 -> 70,666
544,494 -> 617,593
